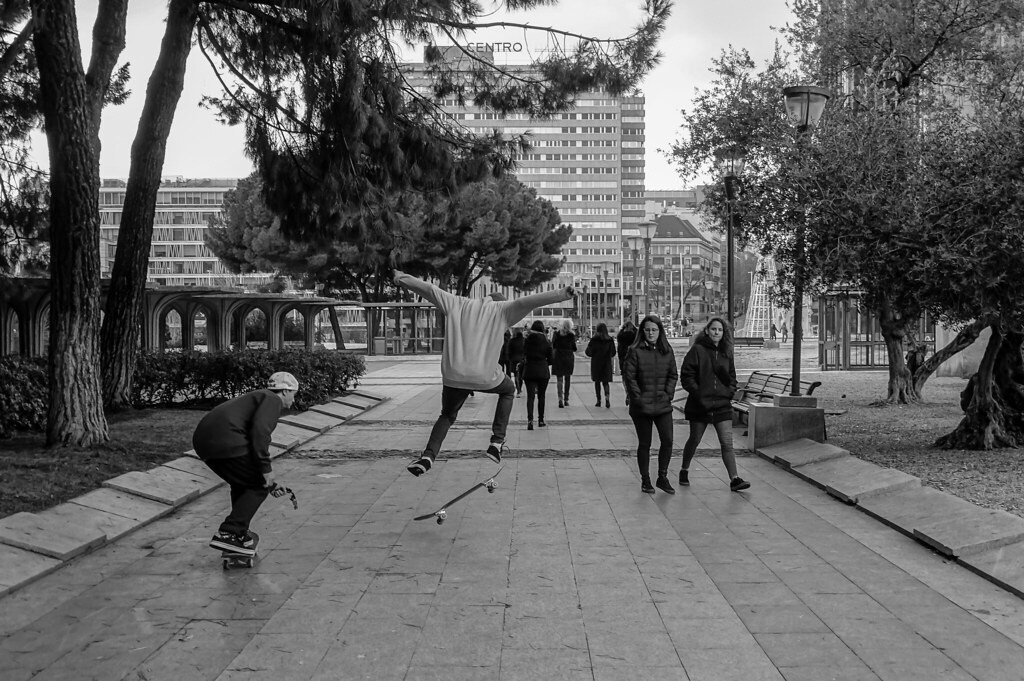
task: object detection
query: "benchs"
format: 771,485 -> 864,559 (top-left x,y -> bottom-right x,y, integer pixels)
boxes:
734,337 -> 764,348
729,369 -> 822,435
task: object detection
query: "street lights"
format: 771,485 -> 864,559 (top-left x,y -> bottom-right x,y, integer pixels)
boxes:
637,220 -> 658,318
601,261 -> 611,323
593,266 -> 602,323
779,87 -> 833,403
714,145 -> 756,334
626,236 -> 642,324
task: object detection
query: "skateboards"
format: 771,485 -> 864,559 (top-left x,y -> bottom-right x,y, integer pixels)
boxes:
221,531 -> 260,571
413,462 -> 507,526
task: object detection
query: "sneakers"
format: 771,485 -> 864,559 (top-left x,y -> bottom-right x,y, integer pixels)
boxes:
407,454 -> 432,476
209,532 -> 255,555
486,444 -> 510,463
237,533 -> 254,547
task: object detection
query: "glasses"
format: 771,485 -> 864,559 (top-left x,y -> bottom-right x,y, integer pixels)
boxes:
643,328 -> 659,334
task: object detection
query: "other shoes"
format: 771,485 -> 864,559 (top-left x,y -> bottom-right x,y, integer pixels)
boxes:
679,468 -> 689,485
626,394 -> 630,405
528,422 -> 533,430
656,476 -> 675,493
595,400 -> 601,407
605,397 -> 610,408
564,402 -> 569,406
730,477 -> 750,490
559,400 -> 563,408
641,477 -> 655,493
539,422 -> 545,426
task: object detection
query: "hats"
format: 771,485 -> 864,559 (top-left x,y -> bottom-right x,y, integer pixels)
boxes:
267,372 -> 299,391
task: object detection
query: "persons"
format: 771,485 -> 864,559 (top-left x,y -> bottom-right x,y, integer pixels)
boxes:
905,345 -> 928,395
780,322 -> 788,343
623,315 -> 679,494
551,319 -> 577,408
522,320 -> 553,430
506,332 -> 523,398
679,318 -> 751,491
769,324 -> 780,340
192,372 -> 299,555
681,317 -> 688,337
585,323 -> 616,409
498,329 -> 511,371
617,320 -> 638,406
390,269 -> 579,478
